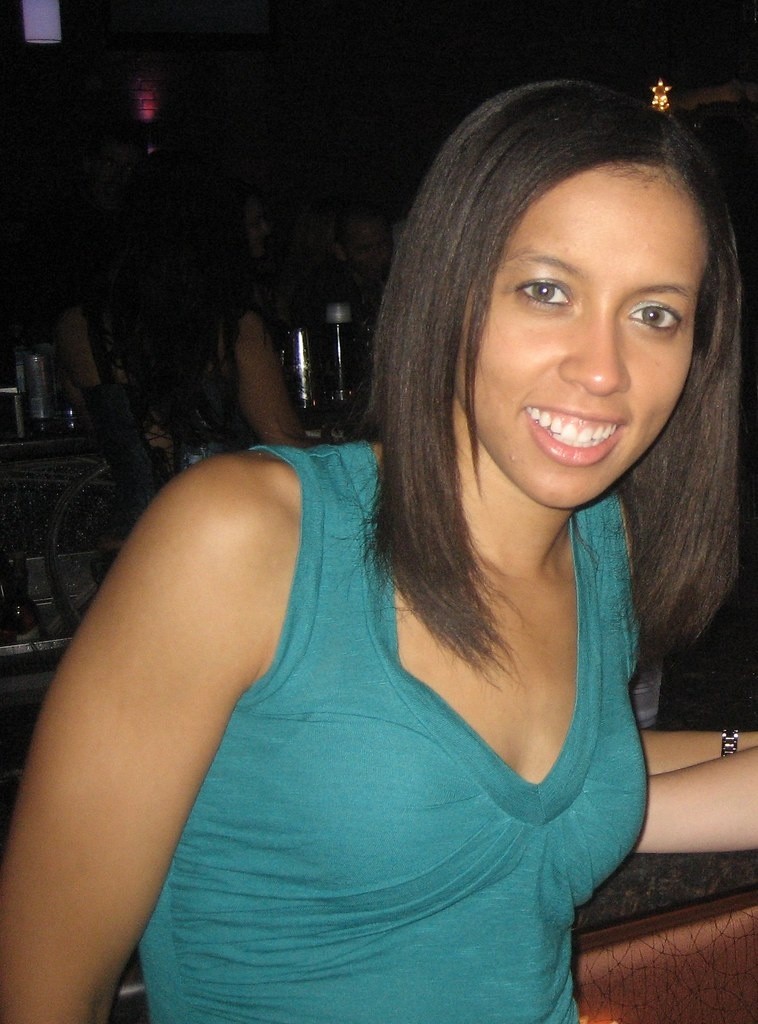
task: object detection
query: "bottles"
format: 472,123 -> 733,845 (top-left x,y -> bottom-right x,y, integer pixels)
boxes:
323,301 -> 358,399
0,557 -> 42,645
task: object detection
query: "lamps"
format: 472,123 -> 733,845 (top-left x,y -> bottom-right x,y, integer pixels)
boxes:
22,0 -> 61,44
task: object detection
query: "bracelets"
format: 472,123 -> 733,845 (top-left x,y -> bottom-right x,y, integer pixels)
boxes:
721,728 -> 739,757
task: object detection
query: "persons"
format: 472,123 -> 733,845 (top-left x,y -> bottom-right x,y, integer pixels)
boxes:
0,79 -> 758,1024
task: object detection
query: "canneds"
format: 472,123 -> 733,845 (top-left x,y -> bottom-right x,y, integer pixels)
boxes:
23,352 -> 56,420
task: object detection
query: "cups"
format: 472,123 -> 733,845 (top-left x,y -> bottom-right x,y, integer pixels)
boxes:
630,655 -> 661,729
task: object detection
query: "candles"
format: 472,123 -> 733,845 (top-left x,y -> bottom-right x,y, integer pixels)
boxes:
326,302 -> 352,323
